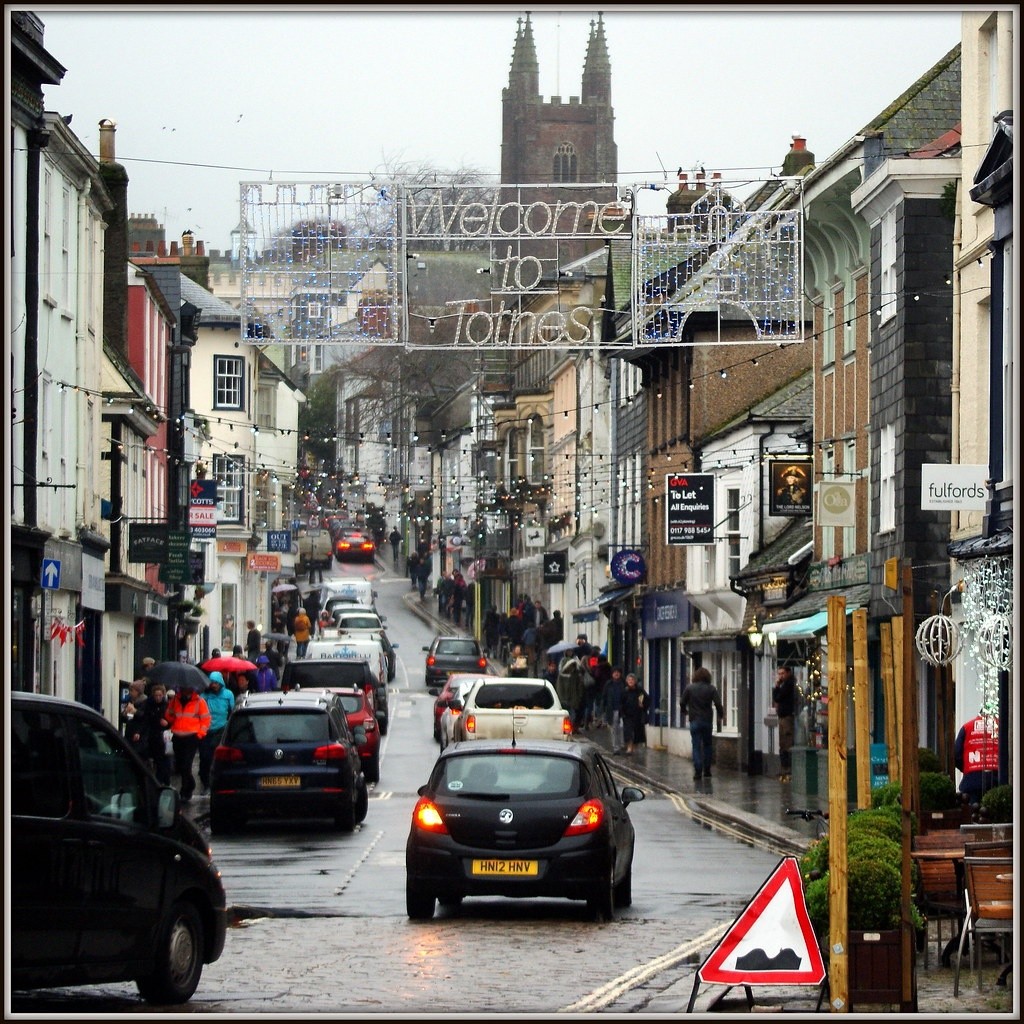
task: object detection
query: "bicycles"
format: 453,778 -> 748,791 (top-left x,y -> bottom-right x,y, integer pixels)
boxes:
786,809 -> 857,841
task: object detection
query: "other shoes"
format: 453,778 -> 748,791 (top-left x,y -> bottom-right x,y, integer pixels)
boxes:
611,751 -> 620,756
181,782 -> 195,801
568,716 -> 603,734
693,767 -> 702,780
703,765 -> 712,777
779,774 -> 793,784
620,744 -> 627,750
200,776 -> 211,795
625,751 -> 632,757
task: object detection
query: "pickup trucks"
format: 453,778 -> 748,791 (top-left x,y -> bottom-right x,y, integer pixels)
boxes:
449,677 -> 572,742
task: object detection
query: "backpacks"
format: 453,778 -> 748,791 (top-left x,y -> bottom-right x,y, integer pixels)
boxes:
296,619 -> 307,632
784,679 -> 806,716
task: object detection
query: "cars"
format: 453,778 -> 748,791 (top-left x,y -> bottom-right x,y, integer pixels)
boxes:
321,509 -> 364,553
335,532 -> 375,563
422,636 -> 500,755
406,740 -> 645,921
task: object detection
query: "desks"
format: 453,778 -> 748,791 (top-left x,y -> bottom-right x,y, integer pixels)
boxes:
911,848 -> 974,970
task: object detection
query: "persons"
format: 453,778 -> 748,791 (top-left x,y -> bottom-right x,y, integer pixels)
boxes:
773,666 -> 799,781
389,526 -> 403,560
119,679 -> 211,801
415,559 -> 431,600
137,657 -> 154,685
211,649 -> 221,659
680,667 -> 722,780
954,706 -> 1000,793
556,635 -> 650,755
407,551 -> 418,588
437,570 -> 475,627
228,621 -> 282,696
271,577 -> 333,660
482,593 -> 563,678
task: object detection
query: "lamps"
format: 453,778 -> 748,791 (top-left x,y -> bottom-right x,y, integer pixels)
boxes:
746,615 -> 764,664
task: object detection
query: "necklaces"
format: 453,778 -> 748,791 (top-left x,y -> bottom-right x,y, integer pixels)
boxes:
197,671 -> 235,795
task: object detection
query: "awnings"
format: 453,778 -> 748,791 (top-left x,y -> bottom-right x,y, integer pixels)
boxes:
778,608 -> 858,640
570,586 -> 629,624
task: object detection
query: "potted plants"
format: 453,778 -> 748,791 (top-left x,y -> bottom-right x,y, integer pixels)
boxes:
811,862 -> 919,1014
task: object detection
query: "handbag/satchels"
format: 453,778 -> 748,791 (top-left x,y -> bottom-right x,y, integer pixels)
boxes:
162,720 -> 175,756
578,659 -> 595,689
638,688 -> 644,707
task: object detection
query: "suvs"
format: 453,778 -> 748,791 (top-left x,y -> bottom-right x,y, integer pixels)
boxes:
210,696 -> 368,836
237,576 -> 400,783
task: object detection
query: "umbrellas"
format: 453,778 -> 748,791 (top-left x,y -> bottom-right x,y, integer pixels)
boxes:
548,641 -> 580,657
263,632 -> 294,651
151,662 -> 211,689
202,657 -> 257,682
272,584 -> 298,592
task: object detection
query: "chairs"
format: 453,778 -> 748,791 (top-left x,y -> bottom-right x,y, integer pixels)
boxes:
545,760 -> 573,783
911,824 -> 1013,997
471,762 -> 497,781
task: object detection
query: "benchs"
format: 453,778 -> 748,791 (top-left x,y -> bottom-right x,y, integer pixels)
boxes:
459,779 -> 569,792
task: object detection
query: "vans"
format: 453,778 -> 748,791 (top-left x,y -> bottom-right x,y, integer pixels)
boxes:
11,691 -> 226,1006
298,530 -> 334,570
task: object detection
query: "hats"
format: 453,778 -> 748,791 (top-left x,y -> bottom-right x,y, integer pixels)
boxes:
129,679 -> 146,695
143,657 -> 154,666
